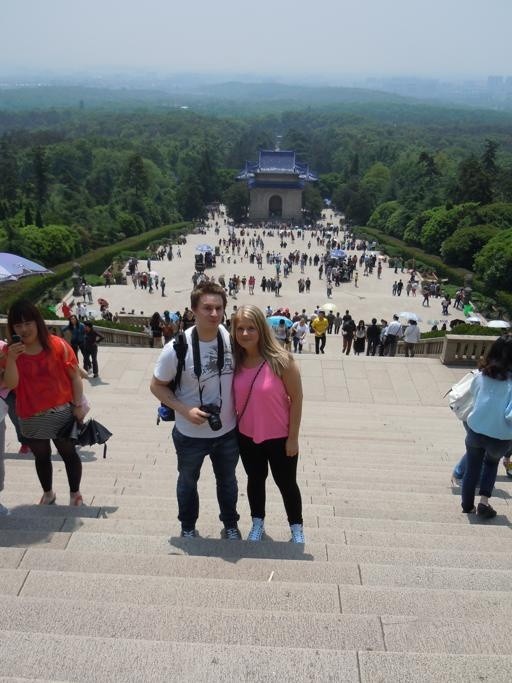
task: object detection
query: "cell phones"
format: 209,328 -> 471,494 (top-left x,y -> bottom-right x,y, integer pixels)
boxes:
13,335 -> 20,344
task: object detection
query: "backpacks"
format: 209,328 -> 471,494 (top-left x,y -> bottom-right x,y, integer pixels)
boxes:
447,368 -> 483,424
156,333 -> 188,424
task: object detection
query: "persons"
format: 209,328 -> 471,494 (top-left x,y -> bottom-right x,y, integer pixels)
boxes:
60,316 -> 104,378
89,312 -> 95,320
99,305 -> 119,322
451,333 -> 512,517
79,200 -> 464,359
1,300 -> 88,505
230,305 -> 306,543
150,282 -> 243,541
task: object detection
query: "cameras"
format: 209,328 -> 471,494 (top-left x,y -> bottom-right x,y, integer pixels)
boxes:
199,403 -> 221,431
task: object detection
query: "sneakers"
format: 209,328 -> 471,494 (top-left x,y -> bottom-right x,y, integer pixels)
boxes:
68,493 -> 83,508
17,442 -> 32,456
503,459 -> 512,477
223,524 -> 242,540
477,502 -> 498,521
450,475 -> 463,487
36,492 -> 57,507
179,525 -> 199,539
289,523 -> 306,544
461,505 -> 476,514
247,517 -> 265,542
